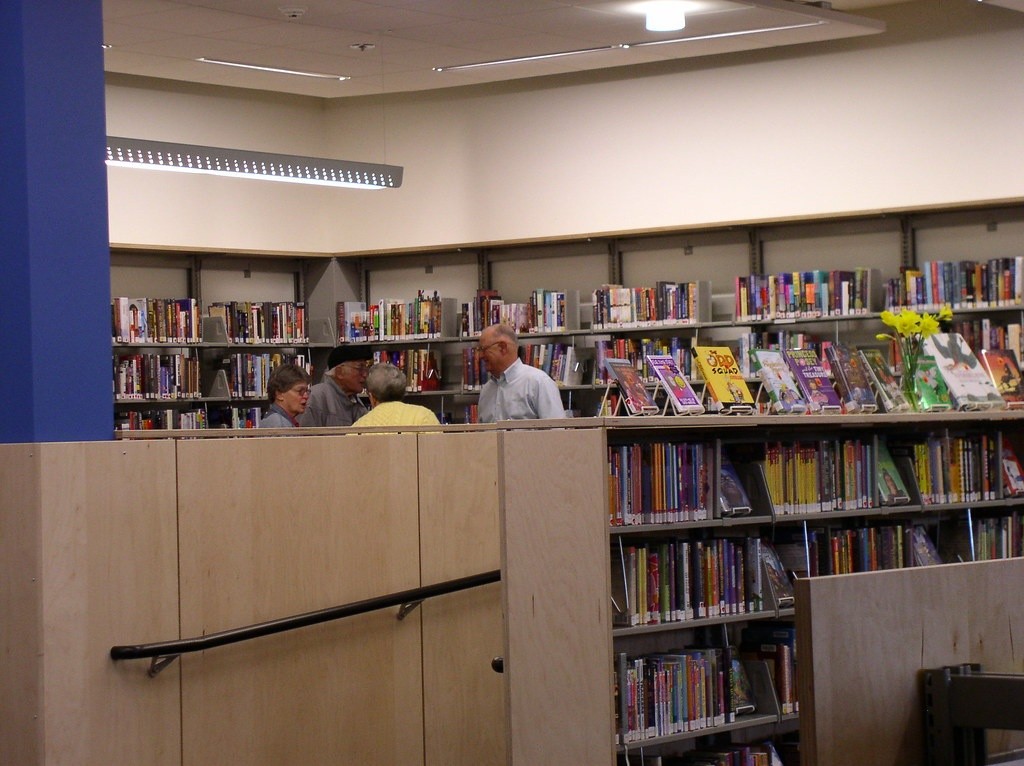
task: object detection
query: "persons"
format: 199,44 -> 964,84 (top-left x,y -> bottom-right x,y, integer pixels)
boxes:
719,473 -> 743,508
477,324 -> 566,432
293,344 -> 371,425
883,469 -> 906,502
258,363 -> 312,427
346,362 -> 442,436
767,563 -> 786,599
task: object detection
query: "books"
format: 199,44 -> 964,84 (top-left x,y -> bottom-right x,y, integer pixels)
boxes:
116,254 -> 1024,766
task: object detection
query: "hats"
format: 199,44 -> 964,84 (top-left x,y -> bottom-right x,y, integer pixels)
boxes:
326,345 -> 374,370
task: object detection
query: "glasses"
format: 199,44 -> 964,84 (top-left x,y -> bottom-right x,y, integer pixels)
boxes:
340,364 -> 370,373
281,383 -> 311,396
476,341 -> 499,352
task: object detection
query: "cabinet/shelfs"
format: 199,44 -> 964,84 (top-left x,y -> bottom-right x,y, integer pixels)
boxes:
105,191 -> 1024,766
